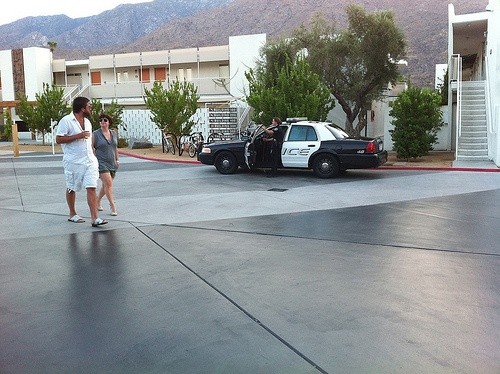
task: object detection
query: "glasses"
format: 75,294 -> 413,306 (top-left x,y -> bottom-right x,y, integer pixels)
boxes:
99,119 -> 109,122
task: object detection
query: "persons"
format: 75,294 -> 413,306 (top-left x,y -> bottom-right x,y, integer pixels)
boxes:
258,117 -> 283,178
92,114 -> 120,216
55,96 -> 107,228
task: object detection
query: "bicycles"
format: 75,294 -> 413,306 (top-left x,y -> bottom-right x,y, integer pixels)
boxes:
191,132 -> 204,149
178,133 -> 197,158
160,124 -> 175,155
207,132 -> 226,143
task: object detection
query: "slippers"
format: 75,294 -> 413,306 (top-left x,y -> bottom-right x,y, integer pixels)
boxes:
68,215 -> 85,223
92,218 -> 108,227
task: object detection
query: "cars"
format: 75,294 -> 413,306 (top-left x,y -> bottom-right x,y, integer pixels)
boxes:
197,117 -> 388,179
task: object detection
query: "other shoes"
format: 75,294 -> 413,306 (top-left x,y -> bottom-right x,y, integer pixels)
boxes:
98,206 -> 104,211
267,173 -> 276,177
110,209 -> 117,216
